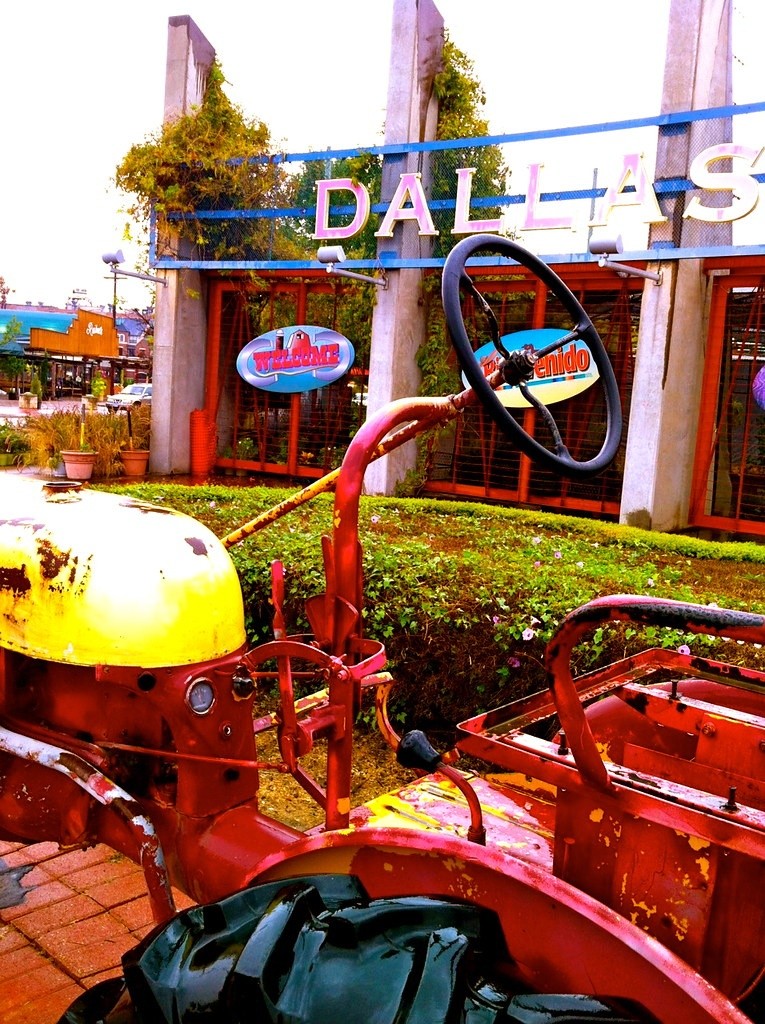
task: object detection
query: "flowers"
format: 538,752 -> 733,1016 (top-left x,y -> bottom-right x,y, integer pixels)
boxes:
236,437 -> 260,460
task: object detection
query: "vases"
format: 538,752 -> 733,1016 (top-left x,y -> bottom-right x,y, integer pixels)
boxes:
236,469 -> 248,477
119,450 -> 151,475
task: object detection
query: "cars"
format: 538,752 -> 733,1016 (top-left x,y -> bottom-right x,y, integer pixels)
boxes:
106,383 -> 152,415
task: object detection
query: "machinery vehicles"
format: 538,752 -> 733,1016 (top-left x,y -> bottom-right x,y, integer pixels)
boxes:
0,234 -> 763,1024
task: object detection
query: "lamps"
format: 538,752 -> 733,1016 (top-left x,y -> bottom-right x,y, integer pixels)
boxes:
589,233 -> 662,286
102,249 -> 169,288
317,245 -> 389,290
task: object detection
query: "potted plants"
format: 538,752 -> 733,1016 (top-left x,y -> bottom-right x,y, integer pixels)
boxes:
91,374 -> 107,401
223,446 -> 233,475
30,373 -> 43,409
0,420 -> 32,466
60,443 -> 99,479
